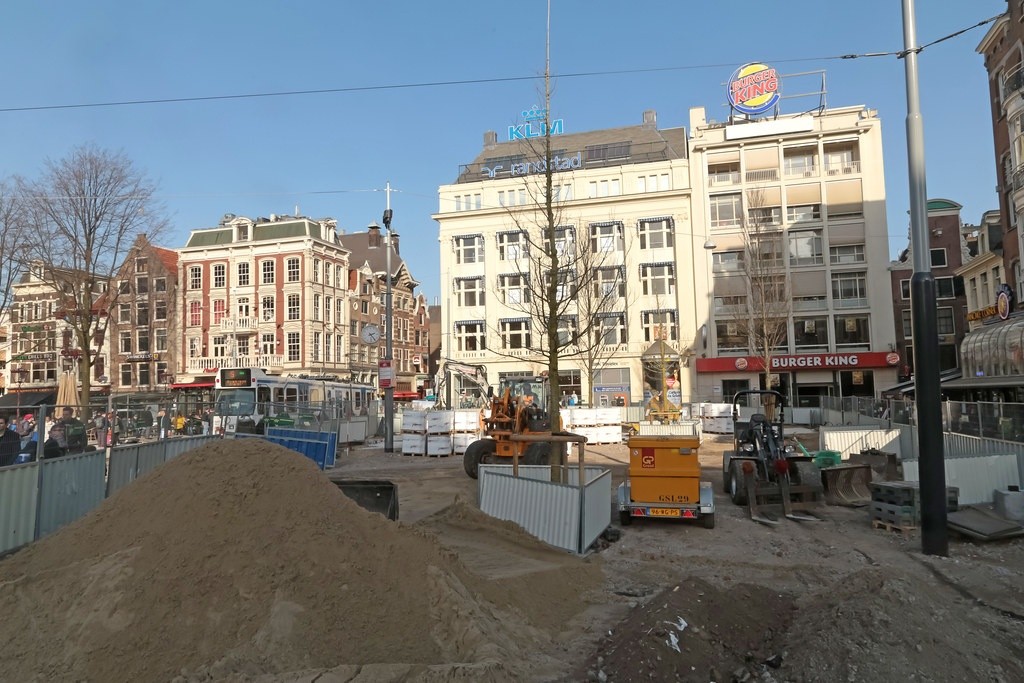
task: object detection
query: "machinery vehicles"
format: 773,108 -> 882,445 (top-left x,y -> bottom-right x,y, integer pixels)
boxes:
464,375 -> 569,480
722,389 -> 825,527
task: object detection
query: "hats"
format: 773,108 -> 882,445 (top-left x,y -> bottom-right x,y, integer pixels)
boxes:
24,414 -> 33,420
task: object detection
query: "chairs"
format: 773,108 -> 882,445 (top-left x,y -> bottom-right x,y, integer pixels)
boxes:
748,414 -> 770,433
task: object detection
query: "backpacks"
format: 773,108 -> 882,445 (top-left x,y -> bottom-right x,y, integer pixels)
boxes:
96,416 -> 104,429
19,421 -> 30,436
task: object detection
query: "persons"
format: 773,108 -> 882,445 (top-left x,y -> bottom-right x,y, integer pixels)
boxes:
0,405 -> 211,467
560,390 -> 579,407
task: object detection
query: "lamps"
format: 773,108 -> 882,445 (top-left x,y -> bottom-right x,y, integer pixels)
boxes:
704,236 -> 717,249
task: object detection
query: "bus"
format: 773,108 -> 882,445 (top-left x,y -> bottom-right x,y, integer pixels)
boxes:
210,367 -> 377,437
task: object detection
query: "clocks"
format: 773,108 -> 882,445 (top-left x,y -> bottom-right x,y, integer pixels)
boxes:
361,325 -> 381,344
